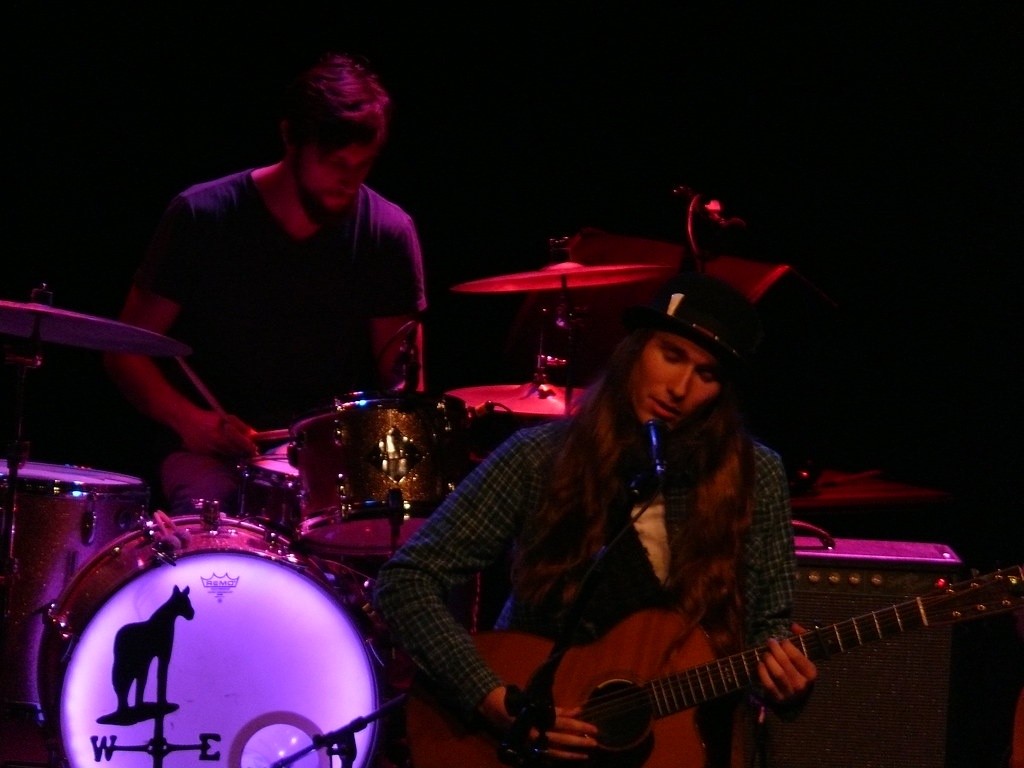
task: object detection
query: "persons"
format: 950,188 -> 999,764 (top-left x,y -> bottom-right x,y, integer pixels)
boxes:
372,269 -> 818,768
103,55 -> 430,515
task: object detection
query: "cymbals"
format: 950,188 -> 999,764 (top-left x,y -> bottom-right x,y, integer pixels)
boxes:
445,383 -> 593,415
451,260 -> 672,293
0,299 -> 194,357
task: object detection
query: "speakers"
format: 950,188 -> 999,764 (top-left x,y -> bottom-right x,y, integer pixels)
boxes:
726,534 -> 960,768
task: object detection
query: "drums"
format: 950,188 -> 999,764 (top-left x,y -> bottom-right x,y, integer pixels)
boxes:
231,454 -> 299,528
35,513 -> 384,768
288,396 -> 466,556
1,455 -> 148,716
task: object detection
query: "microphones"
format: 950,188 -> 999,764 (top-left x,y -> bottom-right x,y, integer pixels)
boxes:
645,415 -> 666,480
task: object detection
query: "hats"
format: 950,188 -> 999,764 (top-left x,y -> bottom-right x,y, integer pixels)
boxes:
619,276 -> 757,381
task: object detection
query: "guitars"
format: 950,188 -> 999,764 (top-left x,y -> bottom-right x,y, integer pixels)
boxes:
399,564 -> 1024,768
